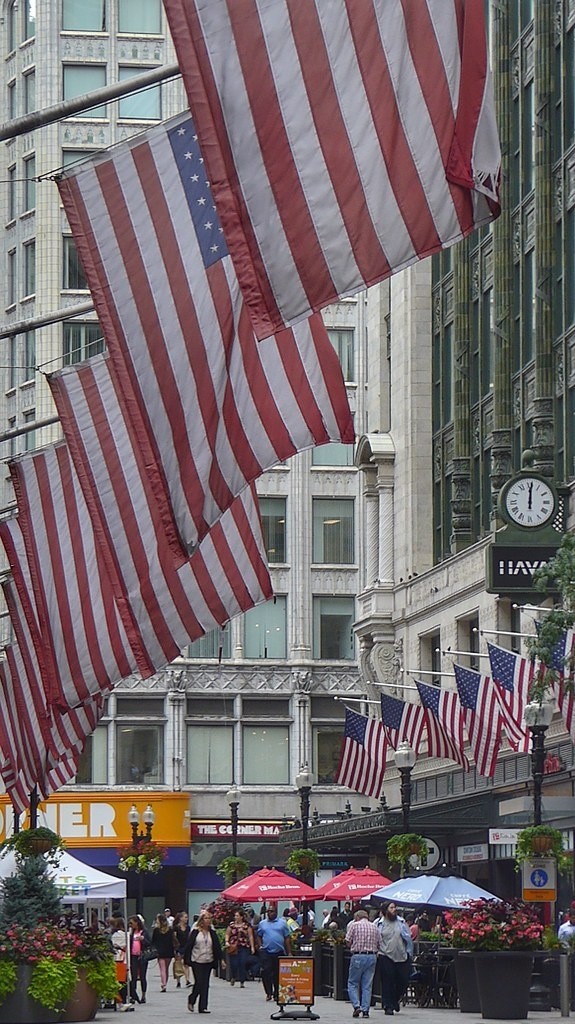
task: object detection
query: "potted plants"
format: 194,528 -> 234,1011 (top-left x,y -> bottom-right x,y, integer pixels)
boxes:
37,911 -> 129,1022
284,848 -> 321,875
542,927 -> 575,955
0,853 -> 67,931
386,833 -> 430,874
0,826 -> 67,869
216,928 -> 227,952
215,856 -> 250,887
514,825 -> 573,877
419,931 -> 450,948
290,929 -> 346,951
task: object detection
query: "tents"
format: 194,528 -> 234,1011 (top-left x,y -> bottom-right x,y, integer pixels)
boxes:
0,833 -> 130,1003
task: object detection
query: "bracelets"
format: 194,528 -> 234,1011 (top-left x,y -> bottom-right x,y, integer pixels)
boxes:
225,941 -> 229,942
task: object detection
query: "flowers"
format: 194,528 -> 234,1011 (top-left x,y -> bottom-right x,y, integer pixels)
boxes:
116,840 -> 168,876
442,897 -> 544,951
200,902 -> 241,925
0,924 -> 82,1013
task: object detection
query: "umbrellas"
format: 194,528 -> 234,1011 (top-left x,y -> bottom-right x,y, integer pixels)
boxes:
316,866 -> 360,913
221,865 -> 325,914
360,871 -> 503,910
323,865 -> 393,907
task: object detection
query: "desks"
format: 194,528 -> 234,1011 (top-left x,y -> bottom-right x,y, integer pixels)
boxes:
311,942 -> 384,1008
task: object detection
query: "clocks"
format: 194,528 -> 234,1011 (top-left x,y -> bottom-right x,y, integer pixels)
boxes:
497,473 -> 559,532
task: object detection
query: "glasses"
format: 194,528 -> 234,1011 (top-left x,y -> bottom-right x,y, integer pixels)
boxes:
266,910 -> 274,913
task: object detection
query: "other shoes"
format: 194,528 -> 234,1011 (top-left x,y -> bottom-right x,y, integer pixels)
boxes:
161,986 -> 167,994
231,979 -> 235,986
140,998 -> 146,1003
240,983 -> 245,988
266,995 -> 272,1001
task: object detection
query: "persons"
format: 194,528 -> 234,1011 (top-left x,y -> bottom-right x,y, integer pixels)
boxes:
346,901 -> 413,1017
557,901 -> 575,947
183,912 -> 226,1014
86,907 -> 200,1003
257,906 -> 293,1001
246,900 -> 446,940
225,908 -> 255,988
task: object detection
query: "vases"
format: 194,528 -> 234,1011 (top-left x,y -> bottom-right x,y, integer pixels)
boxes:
458,951 -> 548,1020
438,948 -> 480,1012
0,965 -> 68,1024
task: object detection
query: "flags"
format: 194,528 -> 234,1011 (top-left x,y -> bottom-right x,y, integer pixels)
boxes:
0,509 -> 108,816
453,661 -> 501,778
54,110 -> 356,562
163,0 -> 503,342
379,692 -> 425,762
414,678 -> 470,774
332,704 -> 386,799
534,619 -> 575,734
486,641 -> 535,756
45,350 -> 276,680
9,438 -> 140,715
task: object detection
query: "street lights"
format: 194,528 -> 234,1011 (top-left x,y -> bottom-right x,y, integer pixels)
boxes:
129,801 -> 154,914
396,737 -> 417,880
523,690 -> 553,1012
226,781 -> 241,884
295,763 -> 315,928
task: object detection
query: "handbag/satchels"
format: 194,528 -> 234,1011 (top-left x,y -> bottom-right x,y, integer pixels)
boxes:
228,944 -> 238,956
141,946 -> 158,961
117,963 -> 126,981
173,961 -> 185,979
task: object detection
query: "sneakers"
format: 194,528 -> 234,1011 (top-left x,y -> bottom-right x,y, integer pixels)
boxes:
353,1009 -> 361,1017
363,1012 -> 369,1018
176,982 -> 181,988
394,1004 -> 400,1012
385,1008 -> 394,1015
186,982 -> 195,988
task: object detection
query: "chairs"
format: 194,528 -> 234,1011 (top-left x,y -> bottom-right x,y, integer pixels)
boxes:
398,961 -> 459,1009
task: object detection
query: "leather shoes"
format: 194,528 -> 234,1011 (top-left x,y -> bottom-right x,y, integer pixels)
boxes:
200,1009 -> 211,1014
189,1004 -> 194,1012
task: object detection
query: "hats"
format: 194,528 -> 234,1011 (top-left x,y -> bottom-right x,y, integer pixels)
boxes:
288,908 -> 298,915
165,909 -> 171,912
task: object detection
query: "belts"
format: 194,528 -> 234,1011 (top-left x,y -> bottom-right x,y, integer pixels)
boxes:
354,951 -> 377,954
